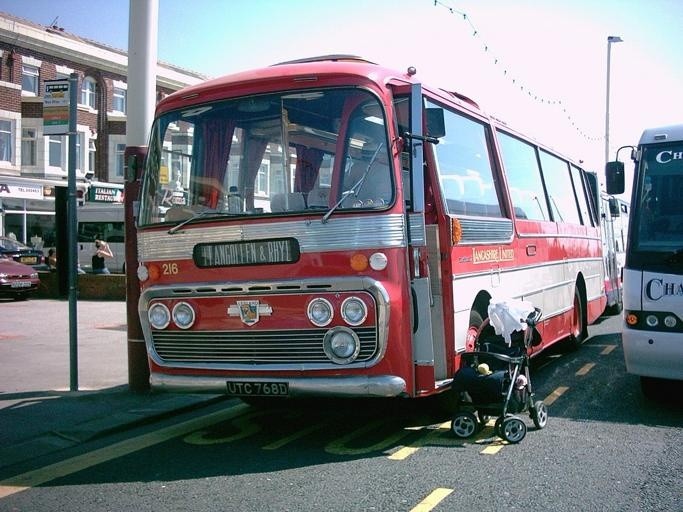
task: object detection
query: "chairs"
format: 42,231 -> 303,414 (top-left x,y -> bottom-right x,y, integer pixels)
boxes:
400,170 -> 506,221
338,160 -> 394,208
307,186 -> 332,210
268,189 -> 303,213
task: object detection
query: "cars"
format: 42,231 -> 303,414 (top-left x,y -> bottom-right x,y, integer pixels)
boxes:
0,236 -> 49,280
0,254 -> 40,300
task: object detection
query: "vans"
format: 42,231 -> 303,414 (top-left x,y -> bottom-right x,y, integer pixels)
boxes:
75,203 -> 172,274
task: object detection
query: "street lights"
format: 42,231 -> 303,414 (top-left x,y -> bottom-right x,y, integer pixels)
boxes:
604,33 -> 625,193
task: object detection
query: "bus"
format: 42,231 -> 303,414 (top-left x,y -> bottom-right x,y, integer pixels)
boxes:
604,122 -> 683,400
124,53 -> 605,410
600,190 -> 629,315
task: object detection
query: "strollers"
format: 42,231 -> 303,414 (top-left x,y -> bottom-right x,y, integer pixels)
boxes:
449,305 -> 548,444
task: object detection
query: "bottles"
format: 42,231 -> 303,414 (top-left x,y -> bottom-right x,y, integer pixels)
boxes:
227,186 -> 241,214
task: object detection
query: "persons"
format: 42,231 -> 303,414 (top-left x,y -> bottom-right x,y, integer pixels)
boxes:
92,240 -> 114,274
48,247 -> 57,271
